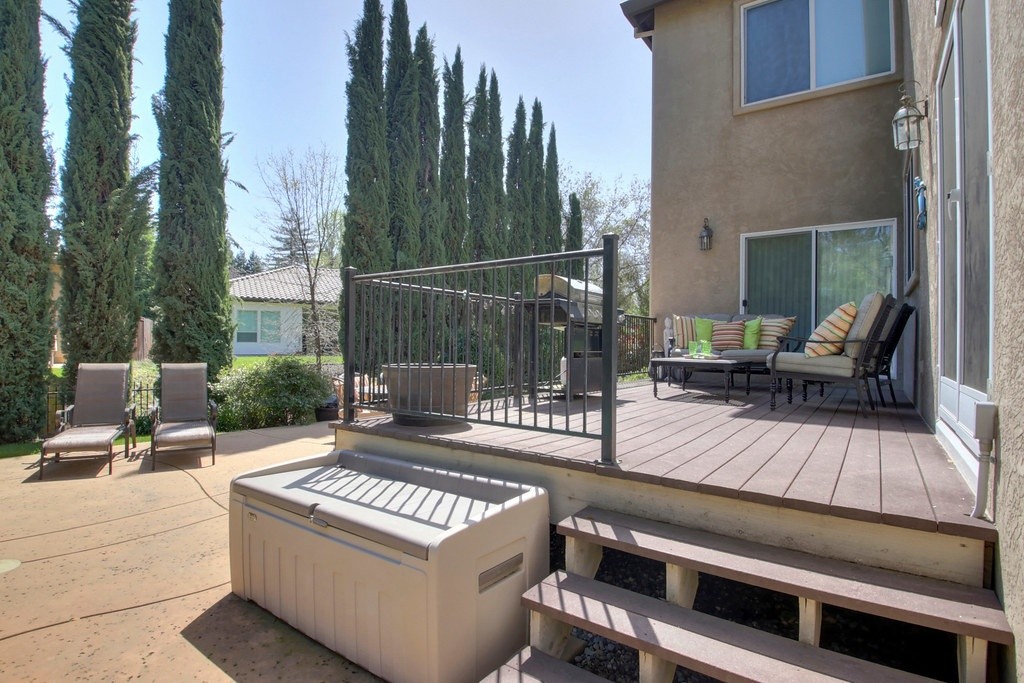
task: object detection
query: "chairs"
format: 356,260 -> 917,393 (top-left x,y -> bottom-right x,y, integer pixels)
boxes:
802,303 -> 917,408
150,362 -> 218,471
40,362 -> 137,476
768,291 -> 898,422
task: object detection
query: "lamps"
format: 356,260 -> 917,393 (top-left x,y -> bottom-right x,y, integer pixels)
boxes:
700,220 -> 714,251
891,80 -> 929,152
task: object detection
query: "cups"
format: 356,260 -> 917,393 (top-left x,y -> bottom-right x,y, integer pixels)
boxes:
702,340 -> 711,355
688,341 -> 697,355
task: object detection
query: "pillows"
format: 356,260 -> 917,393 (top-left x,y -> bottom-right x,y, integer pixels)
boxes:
743,317 -> 763,350
694,315 -> 727,341
804,300 -> 858,358
757,314 -> 798,351
672,314 -> 696,348
711,318 -> 747,350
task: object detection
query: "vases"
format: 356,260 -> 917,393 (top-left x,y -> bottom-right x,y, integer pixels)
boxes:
314,403 -> 339,422
382,363 -> 478,427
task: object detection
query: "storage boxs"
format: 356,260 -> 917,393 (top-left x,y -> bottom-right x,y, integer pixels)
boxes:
224,447 -> 550,683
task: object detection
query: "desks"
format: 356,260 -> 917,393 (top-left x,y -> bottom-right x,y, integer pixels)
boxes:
651,357 -> 753,403
358,385 -> 388,394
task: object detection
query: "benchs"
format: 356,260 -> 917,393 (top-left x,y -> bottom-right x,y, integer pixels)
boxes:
663,313 -> 791,396
